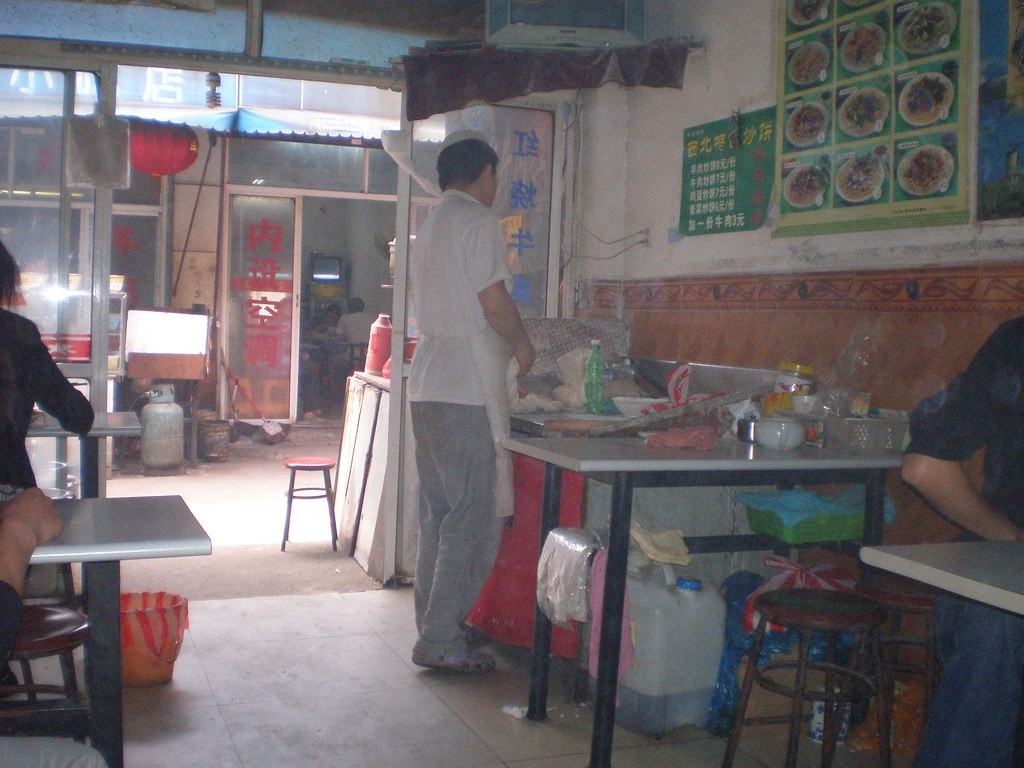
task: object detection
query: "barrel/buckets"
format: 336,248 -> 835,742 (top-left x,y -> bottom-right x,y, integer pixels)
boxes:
587,555 -> 727,738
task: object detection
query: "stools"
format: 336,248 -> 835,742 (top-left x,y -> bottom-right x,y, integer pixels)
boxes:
281,456 -> 338,553
0,605 -> 89,745
40,488 -> 76,596
724,582 -> 937,768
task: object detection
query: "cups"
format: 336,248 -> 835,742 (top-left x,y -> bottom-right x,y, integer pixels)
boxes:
809,687 -> 850,745
737,415 -> 758,442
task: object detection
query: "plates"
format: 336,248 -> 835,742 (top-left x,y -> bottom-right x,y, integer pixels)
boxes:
782,0 -> 957,207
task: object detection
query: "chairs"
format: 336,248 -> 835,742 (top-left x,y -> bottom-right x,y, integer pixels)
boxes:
336,341 -> 368,372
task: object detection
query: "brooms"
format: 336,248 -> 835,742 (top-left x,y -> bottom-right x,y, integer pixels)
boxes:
224,363 -> 286,444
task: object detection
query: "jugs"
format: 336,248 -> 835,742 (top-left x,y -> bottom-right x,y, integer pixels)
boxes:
365,312 -> 392,373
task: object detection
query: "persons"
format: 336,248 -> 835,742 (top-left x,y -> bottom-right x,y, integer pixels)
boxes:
900,313 -> 1024,768
297,295 -> 377,422
405,129 -> 536,674
0,487 -> 110,768
0,242 -> 94,698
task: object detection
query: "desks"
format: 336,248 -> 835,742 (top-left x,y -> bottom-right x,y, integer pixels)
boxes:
860,538 -> 1024,615
28,495 -> 211,768
502,438 -> 907,768
24,413 -> 141,499
334,370 -> 420,579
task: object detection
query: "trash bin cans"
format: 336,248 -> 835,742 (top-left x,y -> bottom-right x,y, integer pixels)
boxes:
120,592 -> 186,684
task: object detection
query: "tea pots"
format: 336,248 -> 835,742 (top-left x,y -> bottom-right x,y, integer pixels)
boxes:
754,414 -> 805,451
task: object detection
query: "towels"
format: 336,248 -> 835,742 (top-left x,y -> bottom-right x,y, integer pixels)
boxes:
631,516 -> 688,564
588,548 -> 636,702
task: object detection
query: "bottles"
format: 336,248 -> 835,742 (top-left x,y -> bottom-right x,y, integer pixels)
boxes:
583,339 -> 604,414
775,361 -> 815,418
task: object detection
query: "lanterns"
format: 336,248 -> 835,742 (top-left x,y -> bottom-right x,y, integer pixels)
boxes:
130,121 -> 200,179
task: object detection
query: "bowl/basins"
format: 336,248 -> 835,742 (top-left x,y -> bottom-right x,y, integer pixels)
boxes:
609,395 -> 670,419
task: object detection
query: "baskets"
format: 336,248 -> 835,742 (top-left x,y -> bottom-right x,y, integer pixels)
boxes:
737,494 -> 866,544
796,413 -> 910,454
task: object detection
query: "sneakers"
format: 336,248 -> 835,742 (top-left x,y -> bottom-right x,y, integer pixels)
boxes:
462,623 -> 477,644
412,645 -> 495,674
